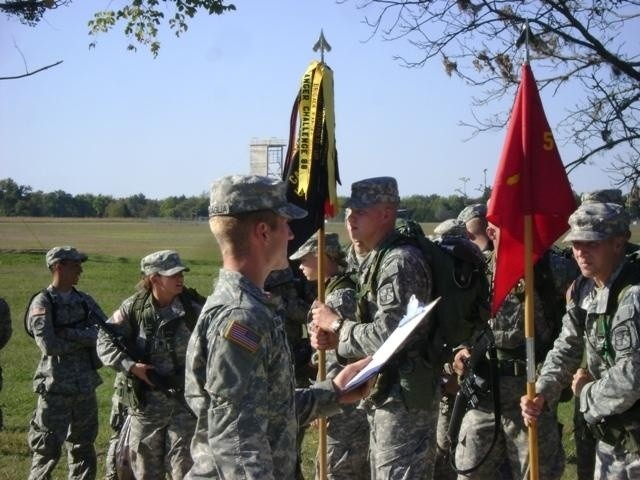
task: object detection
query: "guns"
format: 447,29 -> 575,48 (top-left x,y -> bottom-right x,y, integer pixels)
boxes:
89,307 -> 199,421
437,322 -> 494,464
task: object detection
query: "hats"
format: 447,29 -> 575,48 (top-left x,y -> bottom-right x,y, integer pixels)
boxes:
433,204 -> 486,234
342,177 -> 401,209
141,250 -> 191,277
208,174 -> 308,219
562,189 -> 630,244
45,246 -> 88,268
288,231 -> 342,261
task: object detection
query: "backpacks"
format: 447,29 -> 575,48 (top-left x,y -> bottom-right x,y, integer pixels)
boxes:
357,230 -> 492,350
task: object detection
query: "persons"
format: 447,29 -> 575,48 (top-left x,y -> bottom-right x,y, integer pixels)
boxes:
184,178 -> 639,479
99,249 -> 206,479
26,247 -> 108,480
0,290 -> 12,432
